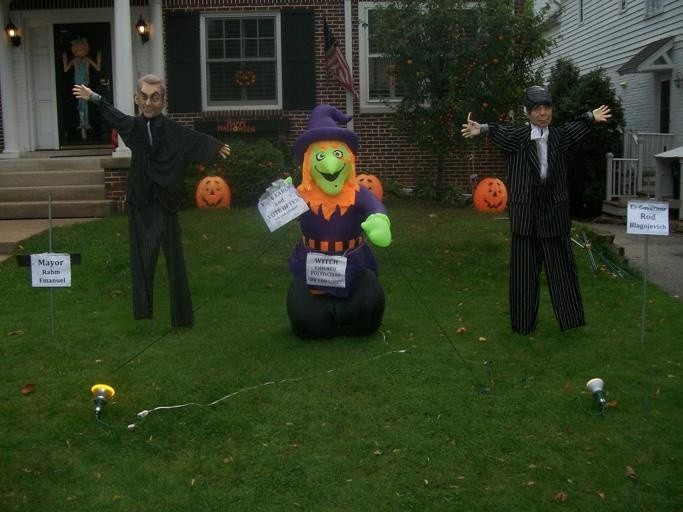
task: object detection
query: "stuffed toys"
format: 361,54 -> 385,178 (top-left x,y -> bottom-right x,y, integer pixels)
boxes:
267,105 -> 393,341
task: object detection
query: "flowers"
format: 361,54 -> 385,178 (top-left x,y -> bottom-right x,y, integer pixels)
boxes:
228,65 -> 258,90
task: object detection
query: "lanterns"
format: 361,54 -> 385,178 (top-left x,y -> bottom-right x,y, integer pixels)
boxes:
473,178 -> 508,213
357,174 -> 383,201
195,175 -> 230,209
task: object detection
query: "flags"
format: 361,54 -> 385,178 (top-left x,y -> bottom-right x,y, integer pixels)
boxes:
324,18 -> 359,97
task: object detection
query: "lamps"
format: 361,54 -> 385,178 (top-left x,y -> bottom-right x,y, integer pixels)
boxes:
585,374 -> 607,413
87,378 -> 116,417
134,15 -> 148,44
3,18 -> 20,47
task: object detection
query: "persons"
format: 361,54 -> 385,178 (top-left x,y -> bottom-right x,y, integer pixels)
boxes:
460,86 -> 613,336
72,75 -> 231,327
61,38 -> 101,139
